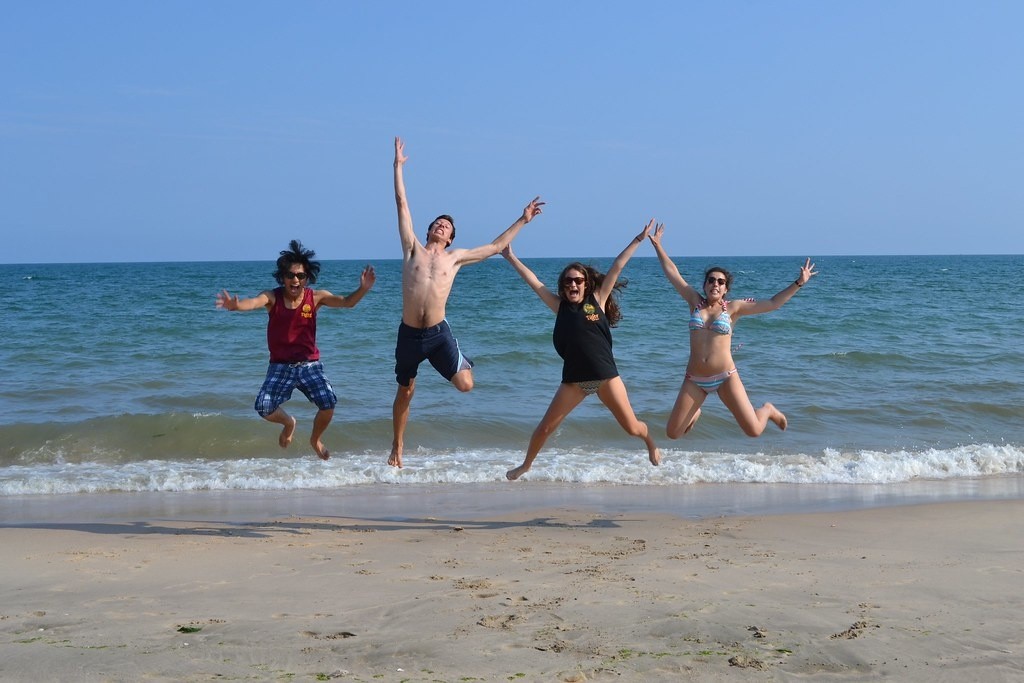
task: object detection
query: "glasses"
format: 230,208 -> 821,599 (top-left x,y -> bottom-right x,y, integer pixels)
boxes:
284,272 -> 308,280
564,277 -> 585,285
707,277 -> 727,285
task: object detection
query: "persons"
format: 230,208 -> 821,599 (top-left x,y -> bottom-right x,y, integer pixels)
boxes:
387,134 -> 547,469
499,217 -> 659,481
215,238 -> 376,461
647,222 -> 818,440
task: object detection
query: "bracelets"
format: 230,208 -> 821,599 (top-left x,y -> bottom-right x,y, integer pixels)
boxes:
636,236 -> 642,243
795,280 -> 802,287
